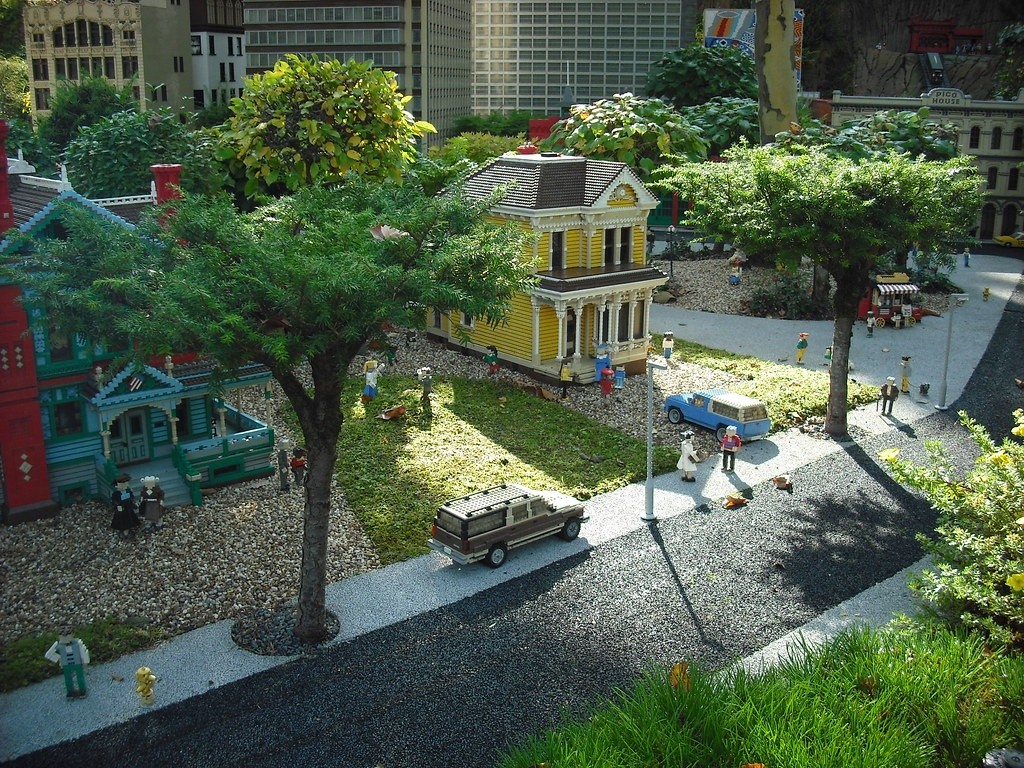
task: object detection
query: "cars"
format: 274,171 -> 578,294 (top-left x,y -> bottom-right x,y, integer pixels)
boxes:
992,232 -> 1024,248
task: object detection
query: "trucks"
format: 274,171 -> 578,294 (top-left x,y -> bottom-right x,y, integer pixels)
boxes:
857,272 -> 924,328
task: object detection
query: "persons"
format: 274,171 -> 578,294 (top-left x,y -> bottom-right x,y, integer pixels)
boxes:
44,625 -> 92,700
612,364 -> 626,390
900,355 -> 913,394
597,365 -> 614,400
557,359 -> 575,403
867,311 -> 877,338
962,247 -> 971,268
482,345 -> 499,379
139,476 -> 165,532
662,331 -> 675,360
676,431 -> 699,482
108,473 -> 141,539
290,447 -> 309,488
879,376 -> 900,416
982,287 -> 990,302
795,332 -> 809,365
720,426 -> 741,474
594,344 -> 611,388
276,439 -> 291,491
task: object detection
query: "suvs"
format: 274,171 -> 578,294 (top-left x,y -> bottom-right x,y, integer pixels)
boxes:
664,388 -> 772,443
426,483 -> 590,569
